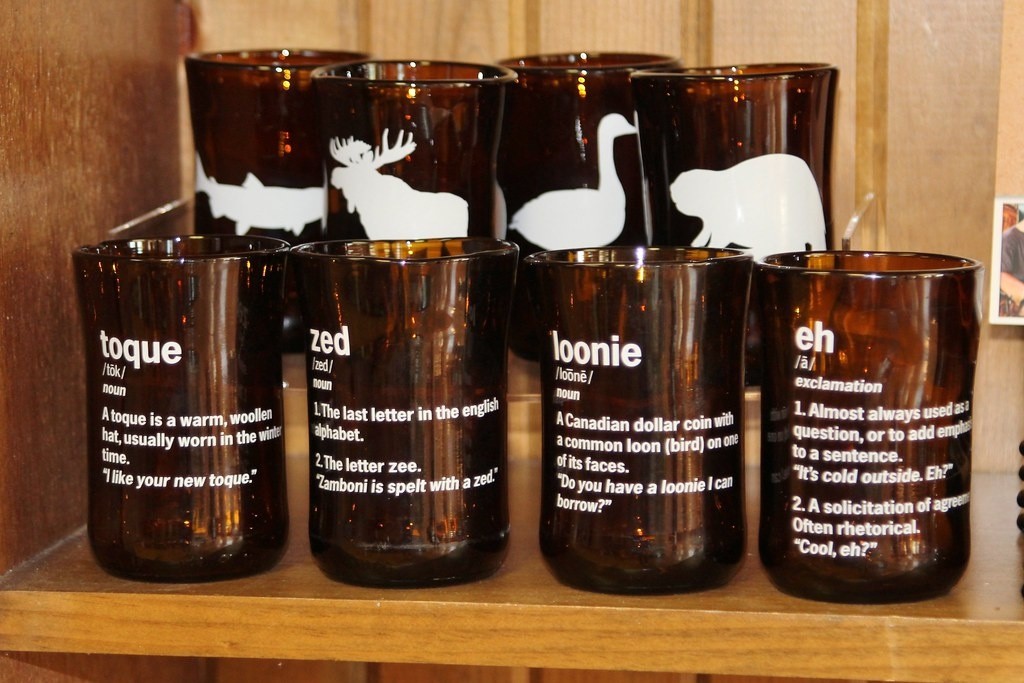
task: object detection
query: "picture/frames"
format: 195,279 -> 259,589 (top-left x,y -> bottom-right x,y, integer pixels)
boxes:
988,197 -> 1024,326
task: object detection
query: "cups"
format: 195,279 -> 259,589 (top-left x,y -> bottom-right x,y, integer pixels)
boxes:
184,48 -> 372,355
628,61 -> 842,387
498,51 -> 682,363
524,245 -> 754,595
70,232 -> 292,584
752,249 -> 981,605
289,236 -> 520,590
308,59 -> 518,243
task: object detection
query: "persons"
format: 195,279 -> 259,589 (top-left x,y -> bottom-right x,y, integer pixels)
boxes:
999,218 -> 1024,319
